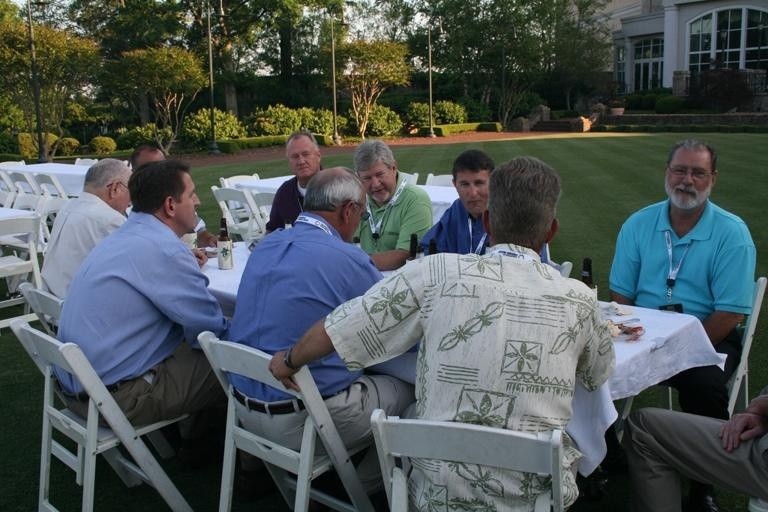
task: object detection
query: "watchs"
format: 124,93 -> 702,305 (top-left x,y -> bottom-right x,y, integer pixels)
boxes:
283,346 -> 296,370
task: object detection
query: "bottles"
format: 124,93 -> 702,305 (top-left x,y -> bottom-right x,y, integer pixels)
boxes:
579,256 -> 597,303
181,229 -> 198,252
353,236 -> 361,249
283,218 -> 293,231
216,218 -> 233,270
406,232 -> 436,263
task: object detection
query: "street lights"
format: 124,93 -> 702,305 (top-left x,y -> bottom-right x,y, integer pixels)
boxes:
427,16 -> 445,139
26,1 -> 53,163
208,0 -> 226,156
330,15 -> 347,139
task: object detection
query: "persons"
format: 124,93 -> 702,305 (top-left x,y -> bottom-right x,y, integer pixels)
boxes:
223,167 -> 416,502
271,158 -> 617,511
264,131 -> 324,232
40,158 -> 133,333
55,160 -> 233,469
609,139 -> 757,508
421,150 -> 547,264
624,386 -> 768,512
123,143 -> 218,250
347,140 -> 432,272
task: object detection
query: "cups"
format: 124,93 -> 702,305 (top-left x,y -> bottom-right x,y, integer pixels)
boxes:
247,234 -> 259,255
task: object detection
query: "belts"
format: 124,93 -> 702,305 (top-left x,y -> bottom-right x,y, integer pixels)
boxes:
231,384 -> 306,415
53,378 -> 126,403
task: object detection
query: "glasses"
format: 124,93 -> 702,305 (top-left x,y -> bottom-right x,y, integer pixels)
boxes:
343,200 -> 370,221
667,162 -> 717,178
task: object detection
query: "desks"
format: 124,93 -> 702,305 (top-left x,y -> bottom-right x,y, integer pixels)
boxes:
10,162 -> 90,199
234,174 -> 460,228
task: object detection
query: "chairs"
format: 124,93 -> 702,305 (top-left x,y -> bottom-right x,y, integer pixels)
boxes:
18,284 -> 64,338
370,408 -> 565,510
74,159 -> 132,166
11,320 -> 195,510
1,159 -> 68,200
401,170 -> 455,188
210,173 -> 292,240
617,277 -> 767,444
0,190 -> 66,334
196,330 -> 389,511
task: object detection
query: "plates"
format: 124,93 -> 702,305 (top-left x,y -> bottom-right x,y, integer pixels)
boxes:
608,321 -> 646,342
603,306 -> 633,315
